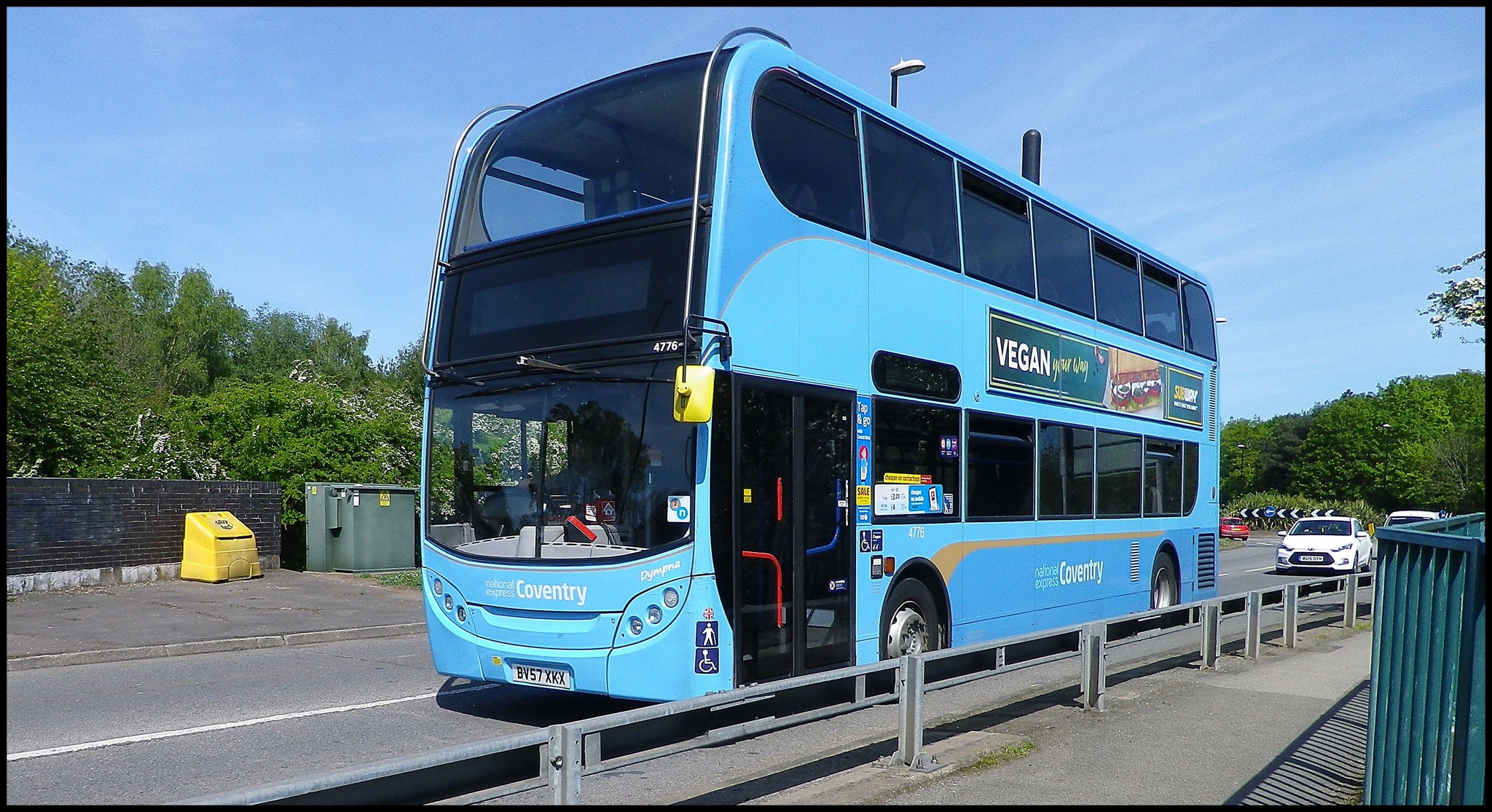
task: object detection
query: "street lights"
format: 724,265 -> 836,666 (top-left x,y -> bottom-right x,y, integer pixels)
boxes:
890,57 -> 927,109
1238,444 -> 1245,499
1383,424 -> 1392,509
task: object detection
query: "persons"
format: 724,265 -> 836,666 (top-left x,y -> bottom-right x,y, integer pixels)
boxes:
1302,523 -> 1317,534
518,434 -> 606,523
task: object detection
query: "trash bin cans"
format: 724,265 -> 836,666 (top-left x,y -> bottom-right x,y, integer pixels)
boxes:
181,511 -> 262,585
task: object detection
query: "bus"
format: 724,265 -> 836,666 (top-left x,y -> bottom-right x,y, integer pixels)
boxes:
417,26 -> 1221,702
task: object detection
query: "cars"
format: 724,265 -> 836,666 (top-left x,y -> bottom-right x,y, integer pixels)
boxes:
1274,516 -> 1373,574
1219,517 -> 1249,541
1383,509 -> 1440,527
